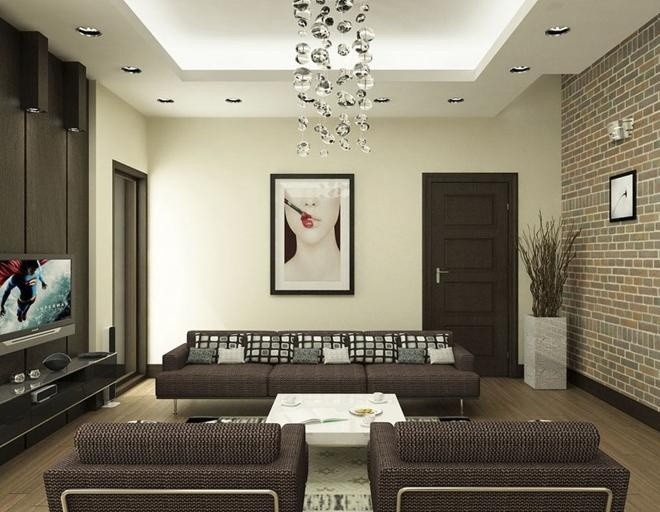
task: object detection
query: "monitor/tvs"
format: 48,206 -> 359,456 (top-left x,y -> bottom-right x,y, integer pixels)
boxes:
0,254 -> 76,357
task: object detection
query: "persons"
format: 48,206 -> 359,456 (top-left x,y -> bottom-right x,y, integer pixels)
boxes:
285,192 -> 341,280
0,263 -> 49,322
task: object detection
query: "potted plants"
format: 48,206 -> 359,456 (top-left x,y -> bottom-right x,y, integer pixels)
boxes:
519,215 -> 579,390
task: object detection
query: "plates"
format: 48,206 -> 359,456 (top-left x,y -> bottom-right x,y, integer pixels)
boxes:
361,418 -> 378,426
349,407 -> 383,415
368,396 -> 387,403
281,398 -> 302,406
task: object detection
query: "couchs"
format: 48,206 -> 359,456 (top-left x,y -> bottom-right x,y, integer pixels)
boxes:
365,420 -> 631,512
154,329 -> 481,416
42,421 -> 310,511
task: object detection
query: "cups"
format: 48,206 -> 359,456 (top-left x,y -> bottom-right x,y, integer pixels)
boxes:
284,396 -> 296,404
365,414 -> 375,424
374,392 -> 384,401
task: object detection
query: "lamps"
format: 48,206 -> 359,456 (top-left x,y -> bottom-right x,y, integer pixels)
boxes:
606,118 -> 635,142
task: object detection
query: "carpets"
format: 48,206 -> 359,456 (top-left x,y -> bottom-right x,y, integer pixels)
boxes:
183,415 -> 472,512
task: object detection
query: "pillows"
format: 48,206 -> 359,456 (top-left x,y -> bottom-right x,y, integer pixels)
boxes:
323,346 -> 351,364
245,333 -> 296,363
216,344 -> 247,365
399,332 -> 450,363
296,333 -> 348,363
186,347 -> 214,364
348,332 -> 399,364
397,347 -> 424,364
292,347 -> 319,365
194,332 -> 246,363
428,347 -> 455,365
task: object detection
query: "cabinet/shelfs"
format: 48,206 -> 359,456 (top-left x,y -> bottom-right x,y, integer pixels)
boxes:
0,350 -> 118,450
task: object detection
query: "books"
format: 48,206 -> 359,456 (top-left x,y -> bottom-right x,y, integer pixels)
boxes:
283,406 -> 349,423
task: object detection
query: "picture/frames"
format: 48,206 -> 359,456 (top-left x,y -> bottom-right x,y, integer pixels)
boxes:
270,173 -> 355,296
609,169 -> 637,223
